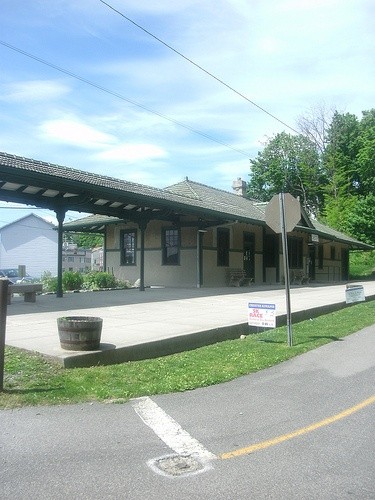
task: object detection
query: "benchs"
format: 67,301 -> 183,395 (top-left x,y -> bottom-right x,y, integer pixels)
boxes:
290,269 -> 313,286
7,284 -> 42,305
226,268 -> 255,288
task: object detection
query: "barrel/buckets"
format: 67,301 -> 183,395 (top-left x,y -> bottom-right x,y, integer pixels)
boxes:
57,315 -> 103,352
